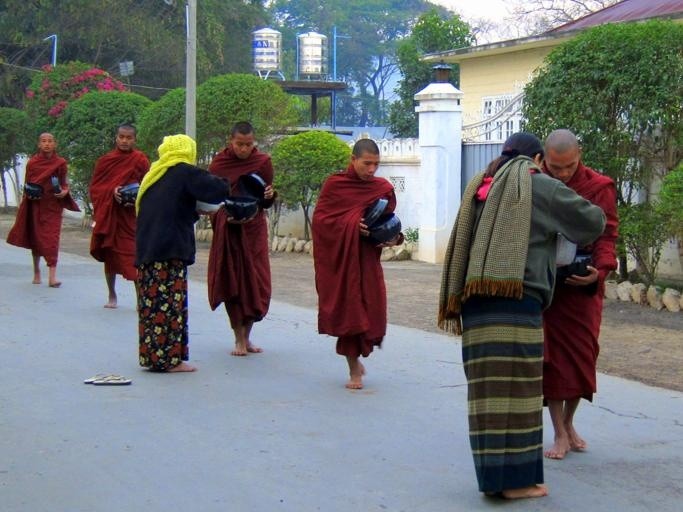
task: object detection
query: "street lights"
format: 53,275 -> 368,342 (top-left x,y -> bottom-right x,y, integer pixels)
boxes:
333,27 -> 352,129
42,34 -> 57,66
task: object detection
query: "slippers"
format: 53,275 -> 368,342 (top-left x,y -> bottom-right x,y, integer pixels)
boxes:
83,372 -> 135,388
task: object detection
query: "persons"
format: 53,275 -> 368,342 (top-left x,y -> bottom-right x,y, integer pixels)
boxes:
538,128 -> 620,462
310,139 -> 405,390
6,131 -> 81,287
135,132 -> 231,375
206,122 -> 279,357
437,131 -> 607,500
88,124 -> 151,310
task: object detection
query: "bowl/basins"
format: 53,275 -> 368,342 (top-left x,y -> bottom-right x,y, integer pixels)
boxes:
555,255 -> 593,288
119,189 -> 140,205
364,213 -> 401,246
224,199 -> 258,220
24,183 -> 44,199
